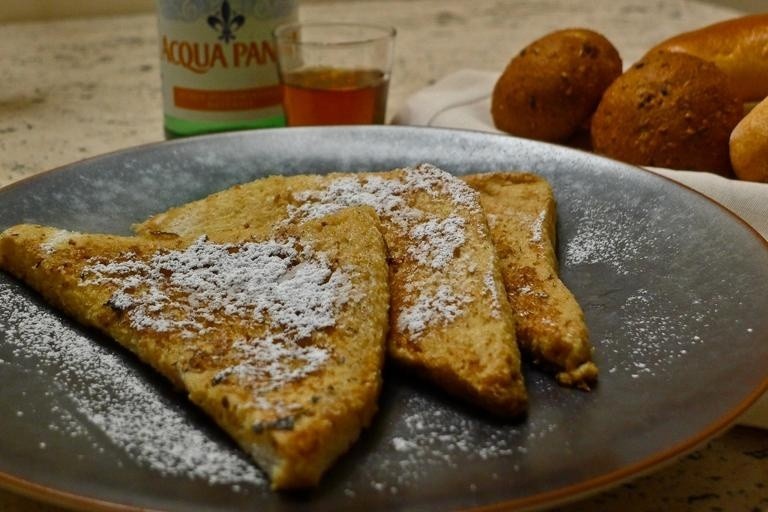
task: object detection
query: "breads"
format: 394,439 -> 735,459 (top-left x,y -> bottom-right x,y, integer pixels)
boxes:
451,168 -> 601,393
0,205 -> 392,490
589,12 -> 768,184
130,163 -> 528,423
490,27 -> 624,145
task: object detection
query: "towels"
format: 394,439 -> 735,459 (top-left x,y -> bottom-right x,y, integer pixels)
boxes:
390,68 -> 768,430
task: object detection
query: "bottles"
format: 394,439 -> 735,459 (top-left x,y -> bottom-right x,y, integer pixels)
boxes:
156,0 -> 301,140
273,22 -> 395,126
